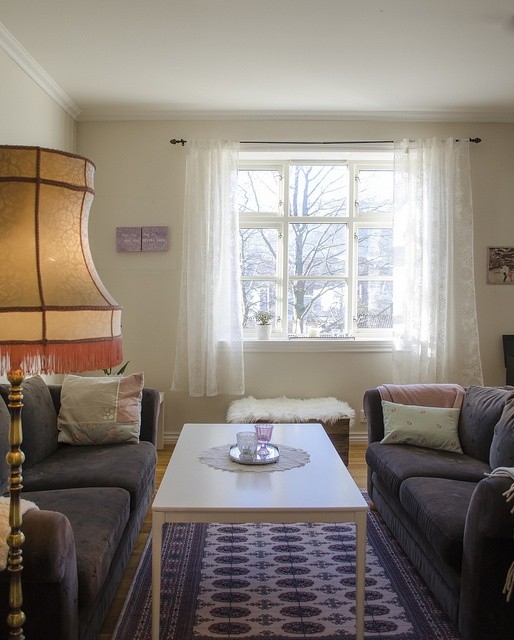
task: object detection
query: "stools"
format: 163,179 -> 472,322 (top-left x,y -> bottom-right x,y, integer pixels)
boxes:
249,418 -> 350,466
157,391 -> 165,450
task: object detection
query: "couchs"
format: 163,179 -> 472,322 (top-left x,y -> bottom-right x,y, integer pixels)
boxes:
364,384 -> 514,639
0,386 -> 160,639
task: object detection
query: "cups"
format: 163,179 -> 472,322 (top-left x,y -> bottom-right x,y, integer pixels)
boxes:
236,431 -> 258,461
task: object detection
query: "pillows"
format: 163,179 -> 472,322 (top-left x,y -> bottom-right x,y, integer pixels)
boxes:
490,389 -> 514,469
0,395 -> 11,487
380,400 -> 463,454
459,385 -> 510,465
57,372 -> 145,445
0,375 -> 60,468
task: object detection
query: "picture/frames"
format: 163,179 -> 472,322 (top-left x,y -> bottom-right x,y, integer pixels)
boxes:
486,246 -> 514,285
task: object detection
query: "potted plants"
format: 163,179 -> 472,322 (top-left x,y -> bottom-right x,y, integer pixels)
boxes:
253,311 -> 276,340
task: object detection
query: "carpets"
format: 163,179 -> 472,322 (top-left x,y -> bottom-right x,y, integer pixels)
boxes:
111,486 -> 458,640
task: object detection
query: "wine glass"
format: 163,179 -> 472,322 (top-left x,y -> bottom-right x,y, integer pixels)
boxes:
254,424 -> 274,456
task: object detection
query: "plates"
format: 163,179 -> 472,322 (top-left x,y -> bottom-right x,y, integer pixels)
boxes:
227,442 -> 280,465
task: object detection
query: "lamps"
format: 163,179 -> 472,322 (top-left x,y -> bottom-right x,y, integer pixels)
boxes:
0,145 -> 123,639
